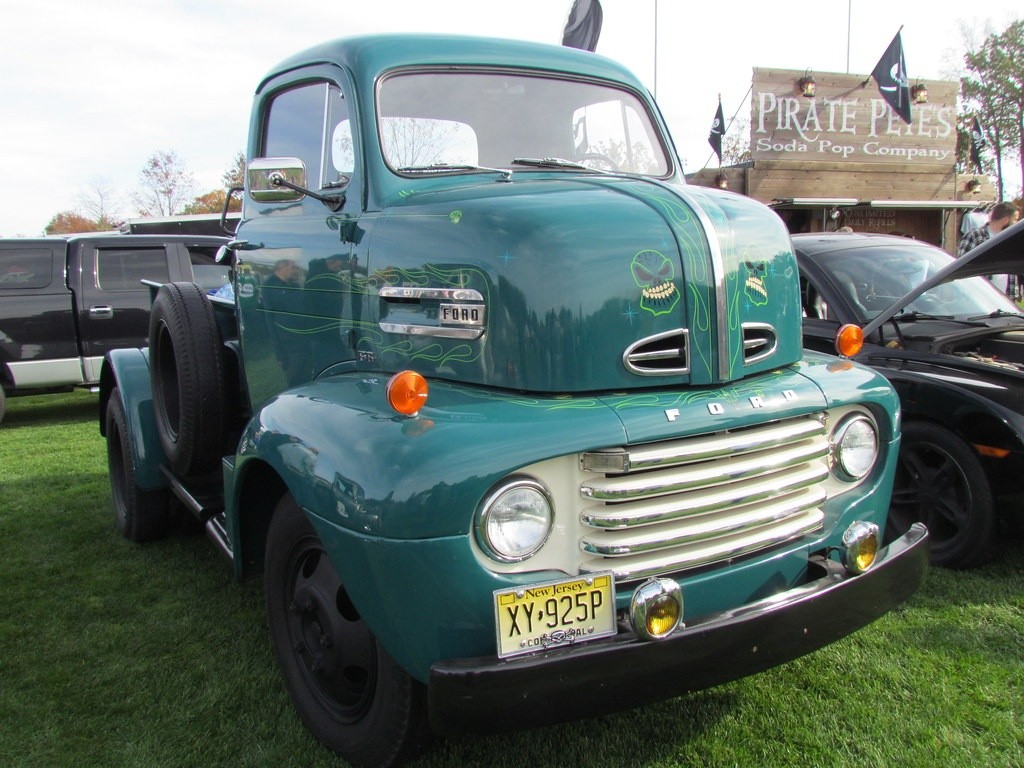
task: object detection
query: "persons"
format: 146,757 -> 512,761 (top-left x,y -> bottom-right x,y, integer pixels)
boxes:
836,225 -> 935,312
266,250 -> 367,387
959,201 -> 1024,304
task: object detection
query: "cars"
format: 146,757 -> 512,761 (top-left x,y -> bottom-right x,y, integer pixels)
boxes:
791,212 -> 1024,575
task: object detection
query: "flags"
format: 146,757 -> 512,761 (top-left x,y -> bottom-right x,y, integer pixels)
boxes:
708,104 -> 725,170
875,34 -> 912,123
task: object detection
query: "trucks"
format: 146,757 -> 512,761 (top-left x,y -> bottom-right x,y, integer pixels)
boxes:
100,33 -> 932,768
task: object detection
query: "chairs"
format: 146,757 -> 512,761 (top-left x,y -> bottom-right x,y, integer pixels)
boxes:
813,270 -> 867,322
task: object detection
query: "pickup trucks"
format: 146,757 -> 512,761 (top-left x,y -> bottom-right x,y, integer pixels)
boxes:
1,235 -> 239,397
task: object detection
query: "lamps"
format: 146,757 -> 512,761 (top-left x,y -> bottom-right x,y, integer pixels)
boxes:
911,74 -> 927,103
800,67 -> 817,97
968,177 -> 982,194
715,166 -> 728,190
